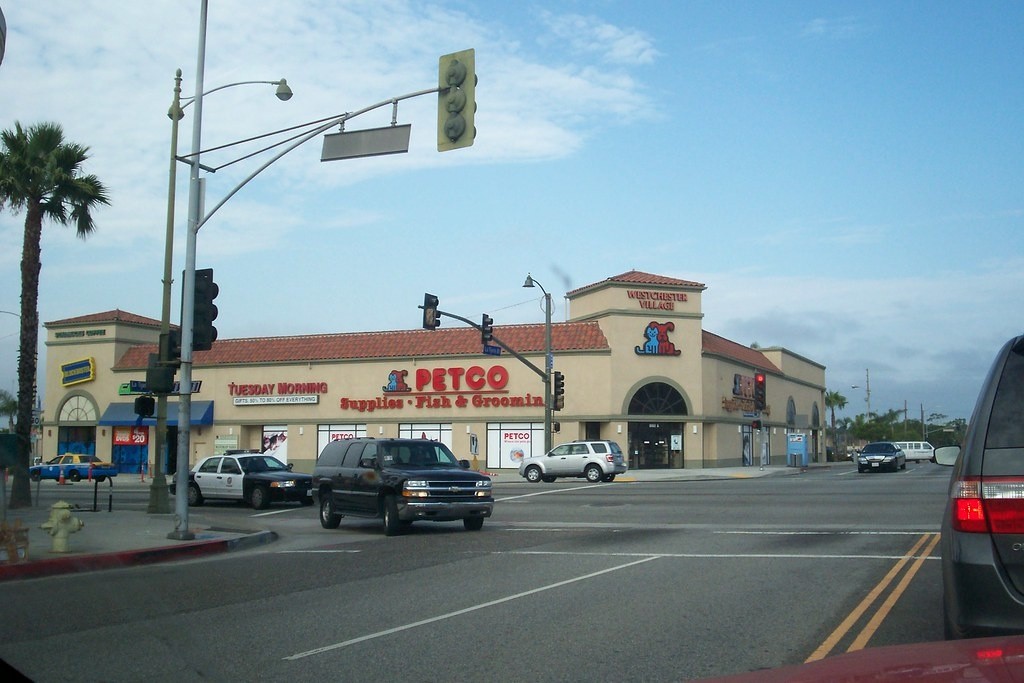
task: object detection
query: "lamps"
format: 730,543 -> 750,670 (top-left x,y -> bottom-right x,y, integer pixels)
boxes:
618,425 -> 622,434
230,428 -> 233,435
466,426 -> 471,434
48,430 -> 51,436
820,431 -> 823,437
300,427 -> 303,435
197,427 -> 201,435
693,425 -> 698,434
738,426 -> 742,433
797,428 -> 800,433
810,430 -> 812,436
773,428 -> 776,434
101,429 -> 105,436
784,428 -> 787,434
379,426 -> 384,434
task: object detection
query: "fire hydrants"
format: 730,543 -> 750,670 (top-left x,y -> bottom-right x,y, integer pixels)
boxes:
41,499 -> 85,553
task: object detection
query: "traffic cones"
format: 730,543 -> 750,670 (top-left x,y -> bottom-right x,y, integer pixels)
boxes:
58,470 -> 65,484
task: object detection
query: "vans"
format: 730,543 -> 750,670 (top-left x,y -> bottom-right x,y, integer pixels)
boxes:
895,441 -> 936,463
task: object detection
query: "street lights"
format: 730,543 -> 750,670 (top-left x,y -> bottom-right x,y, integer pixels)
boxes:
851,384 -> 871,422
147,78 -> 295,513
522,273 -> 552,456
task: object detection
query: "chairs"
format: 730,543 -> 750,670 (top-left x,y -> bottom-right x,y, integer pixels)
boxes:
247,459 -> 267,470
567,447 -> 574,454
408,448 -> 428,462
576,448 -> 581,454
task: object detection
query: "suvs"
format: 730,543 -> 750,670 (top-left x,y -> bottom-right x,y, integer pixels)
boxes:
313,438 -> 496,537
519,440 -> 627,483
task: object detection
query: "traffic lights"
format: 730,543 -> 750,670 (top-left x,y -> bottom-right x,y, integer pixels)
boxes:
554,371 -> 566,411
482,314 -> 494,345
753,372 -> 766,409
424,293 -> 441,330
555,422 -> 560,432
437,49 -> 478,153
753,420 -> 761,429
181,268 -> 219,351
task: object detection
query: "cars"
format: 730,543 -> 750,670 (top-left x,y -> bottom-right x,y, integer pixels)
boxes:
169,449 -> 314,512
29,453 -> 119,482
826,442 -> 906,473
933,334 -> 1024,639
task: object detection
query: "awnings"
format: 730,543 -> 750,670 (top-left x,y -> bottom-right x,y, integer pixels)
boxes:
98,401 -> 213,425
